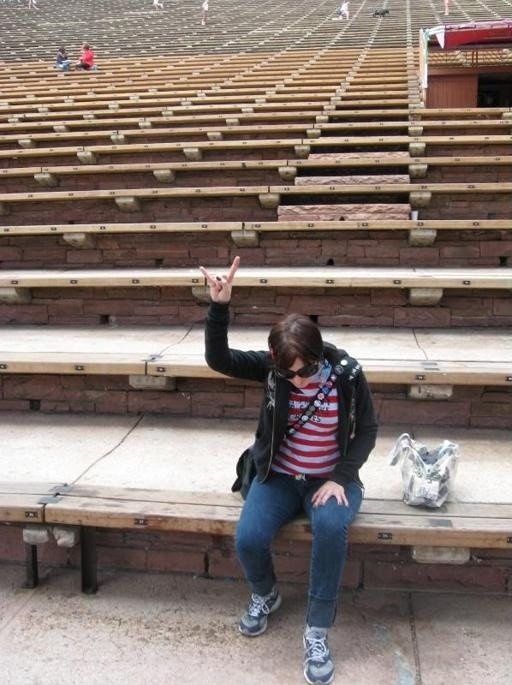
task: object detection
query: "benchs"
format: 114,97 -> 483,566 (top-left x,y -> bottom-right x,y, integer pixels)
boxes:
1,0 -> 508,597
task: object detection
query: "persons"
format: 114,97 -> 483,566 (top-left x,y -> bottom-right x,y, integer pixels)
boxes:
56,46 -> 72,72
201,0 -> 209,25
342,0 -> 351,19
75,41 -> 94,70
442,0 -> 449,14
198,253 -> 379,685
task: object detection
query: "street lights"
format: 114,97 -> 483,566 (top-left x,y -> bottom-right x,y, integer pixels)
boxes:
231,444 -> 257,500
389,432 -> 459,508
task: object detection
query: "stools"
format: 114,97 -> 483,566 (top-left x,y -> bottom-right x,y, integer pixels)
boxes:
272,471 -> 327,481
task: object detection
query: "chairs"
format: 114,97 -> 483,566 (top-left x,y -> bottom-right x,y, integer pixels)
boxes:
238,583 -> 282,637
302,626 -> 335,685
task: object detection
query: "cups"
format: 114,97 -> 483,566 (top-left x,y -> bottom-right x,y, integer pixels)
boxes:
272,360 -> 319,380
